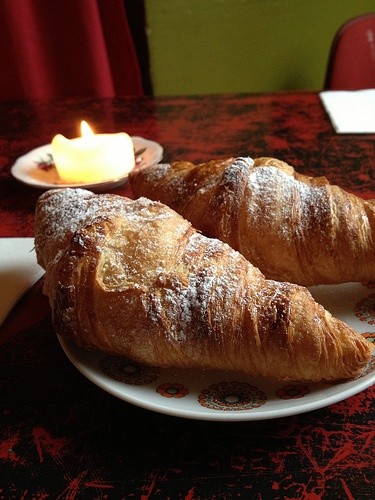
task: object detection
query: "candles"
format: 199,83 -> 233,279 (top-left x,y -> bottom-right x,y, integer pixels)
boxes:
49,119 -> 136,182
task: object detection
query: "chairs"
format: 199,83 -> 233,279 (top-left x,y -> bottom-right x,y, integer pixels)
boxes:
323,10 -> 375,92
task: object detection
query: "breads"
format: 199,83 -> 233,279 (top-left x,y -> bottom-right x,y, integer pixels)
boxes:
34,187 -> 374,378
129,157 -> 375,288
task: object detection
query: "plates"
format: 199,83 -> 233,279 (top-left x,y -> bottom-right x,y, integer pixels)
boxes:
56,280 -> 375,420
12,134 -> 164,193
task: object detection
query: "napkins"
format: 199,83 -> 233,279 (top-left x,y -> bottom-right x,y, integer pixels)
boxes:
0,236 -> 46,324
319,88 -> 375,134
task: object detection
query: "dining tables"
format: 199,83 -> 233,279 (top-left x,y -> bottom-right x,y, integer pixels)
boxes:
0,88 -> 375,500
